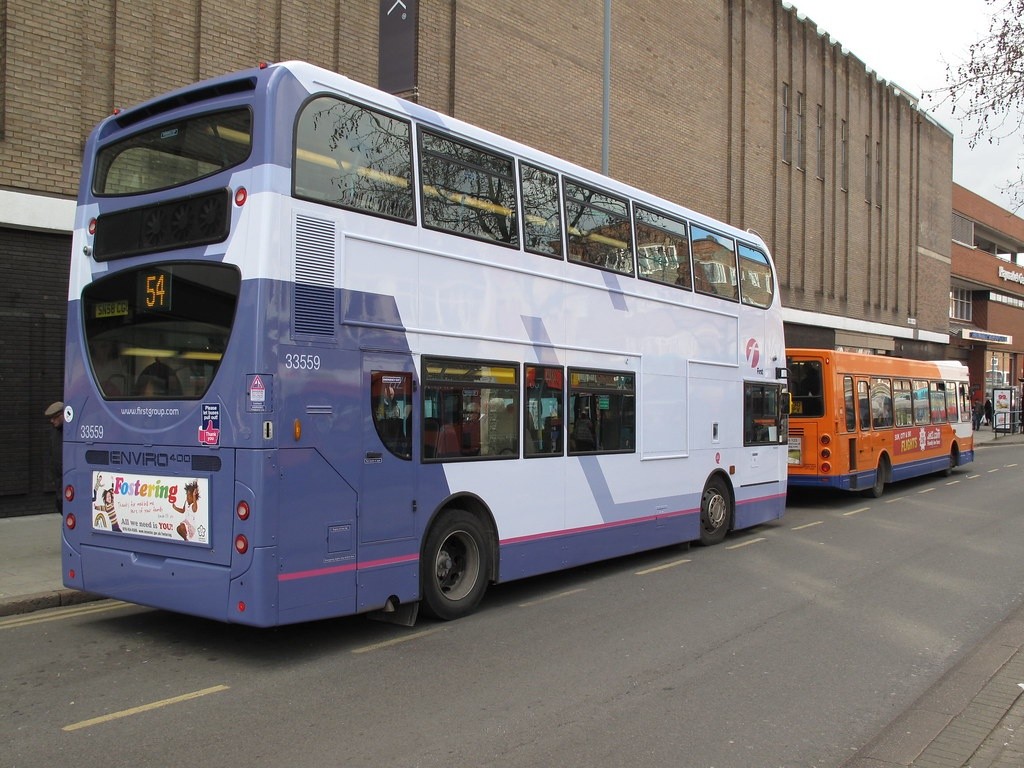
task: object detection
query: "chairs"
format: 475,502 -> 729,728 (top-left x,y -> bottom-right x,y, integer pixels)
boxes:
378,418 -> 479,459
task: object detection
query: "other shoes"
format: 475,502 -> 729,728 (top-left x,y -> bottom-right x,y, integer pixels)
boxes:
972,428 -> 975,430
976,429 -> 979,430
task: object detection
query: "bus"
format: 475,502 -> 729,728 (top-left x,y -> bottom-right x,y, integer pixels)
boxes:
786,348 -> 974,499
59,59 -> 790,627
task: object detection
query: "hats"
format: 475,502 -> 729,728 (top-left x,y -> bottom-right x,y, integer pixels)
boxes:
44,401 -> 64,417
975,398 -> 979,401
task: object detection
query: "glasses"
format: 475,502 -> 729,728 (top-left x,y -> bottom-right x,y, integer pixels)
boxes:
49,413 -> 64,421
465,411 -> 480,415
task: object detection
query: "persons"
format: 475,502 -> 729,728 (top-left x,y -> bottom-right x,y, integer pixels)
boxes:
972,399 -> 984,430
464,402 -> 480,451
384,387 -> 400,417
489,398 -> 512,441
984,400 -> 993,425
44,401 -> 70,517
